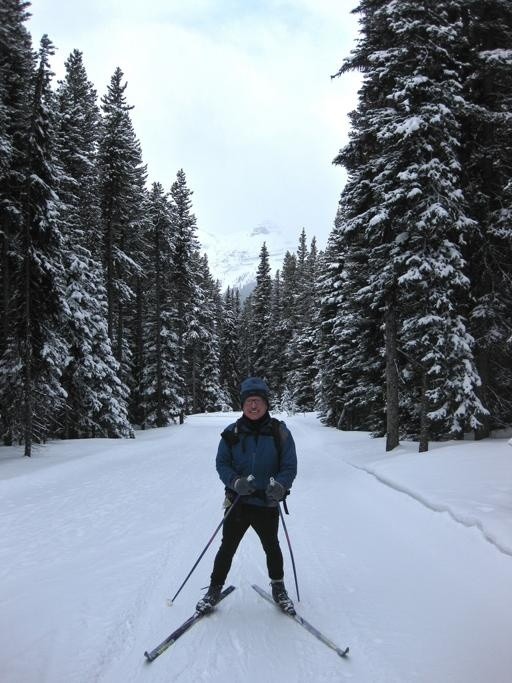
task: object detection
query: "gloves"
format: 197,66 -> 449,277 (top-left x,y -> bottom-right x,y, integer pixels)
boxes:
265,481 -> 286,501
231,475 -> 258,496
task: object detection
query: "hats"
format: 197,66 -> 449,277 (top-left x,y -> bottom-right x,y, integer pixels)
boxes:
240,377 -> 271,409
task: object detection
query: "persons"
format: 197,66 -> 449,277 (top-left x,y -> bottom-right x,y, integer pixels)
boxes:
196,377 -> 297,614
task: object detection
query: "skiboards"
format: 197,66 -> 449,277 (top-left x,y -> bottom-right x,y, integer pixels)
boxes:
144,584 -> 350,661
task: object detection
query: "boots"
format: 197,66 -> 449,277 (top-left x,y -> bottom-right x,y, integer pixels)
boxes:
196,585 -> 222,615
268,578 -> 294,612
